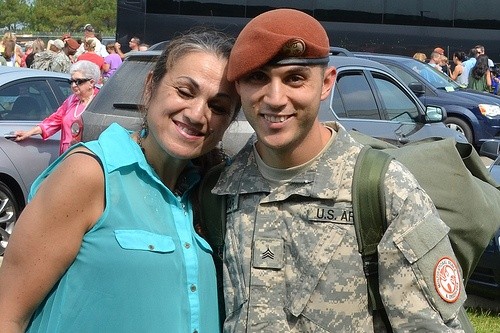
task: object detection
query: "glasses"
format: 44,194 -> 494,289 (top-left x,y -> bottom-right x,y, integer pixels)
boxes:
69,79 -> 92,85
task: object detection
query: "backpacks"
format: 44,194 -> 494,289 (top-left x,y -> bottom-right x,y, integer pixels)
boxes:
30,52 -> 56,72
198,128 -> 500,333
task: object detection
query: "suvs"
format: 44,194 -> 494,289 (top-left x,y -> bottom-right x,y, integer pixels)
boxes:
69,40 -> 467,158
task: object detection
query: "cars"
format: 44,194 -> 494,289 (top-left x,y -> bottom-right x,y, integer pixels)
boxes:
351,52 -> 500,158
0,66 -> 103,256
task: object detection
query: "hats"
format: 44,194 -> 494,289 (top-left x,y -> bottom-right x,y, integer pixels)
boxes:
66,39 -> 80,50
227,9 -> 331,83
83,24 -> 94,31
86,39 -> 96,48
434,48 -> 444,54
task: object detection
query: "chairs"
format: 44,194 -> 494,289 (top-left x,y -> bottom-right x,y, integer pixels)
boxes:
12,96 -> 41,120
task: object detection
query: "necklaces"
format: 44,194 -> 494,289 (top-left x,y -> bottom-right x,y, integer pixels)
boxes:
74,94 -> 93,118
137,132 -> 186,196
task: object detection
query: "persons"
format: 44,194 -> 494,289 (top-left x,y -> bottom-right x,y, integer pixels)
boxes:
412,44 -> 500,94
199,8 -> 476,333
0,23 -> 149,87
0,32 -> 242,333
15,60 -> 103,158
